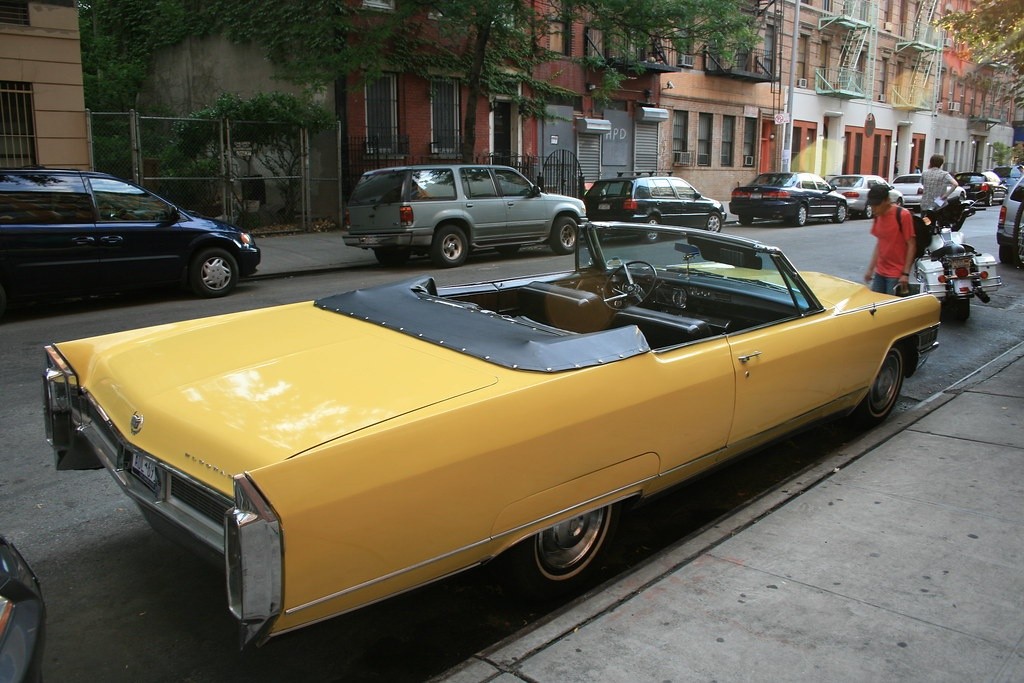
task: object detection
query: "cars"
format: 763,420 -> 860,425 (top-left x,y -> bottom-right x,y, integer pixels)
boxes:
954,171 -> 1008,206
42,220 -> 943,653
729,172 -> 848,227
828,175 -> 906,219
891,175 -> 967,209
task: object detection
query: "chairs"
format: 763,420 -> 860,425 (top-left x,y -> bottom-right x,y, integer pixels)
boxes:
612,306 -> 712,349
519,281 -> 616,334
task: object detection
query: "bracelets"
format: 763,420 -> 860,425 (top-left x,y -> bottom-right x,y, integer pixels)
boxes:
902,272 -> 910,276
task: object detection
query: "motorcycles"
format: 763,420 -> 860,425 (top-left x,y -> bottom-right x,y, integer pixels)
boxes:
915,190 -> 1003,325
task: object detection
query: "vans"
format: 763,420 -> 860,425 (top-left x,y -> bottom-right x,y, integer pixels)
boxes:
0,166 -> 262,314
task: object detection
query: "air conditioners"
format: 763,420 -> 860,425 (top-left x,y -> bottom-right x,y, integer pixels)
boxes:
947,102 -> 960,111
674,152 -> 691,166
744,156 -> 753,166
797,78 -> 807,88
879,94 -> 885,102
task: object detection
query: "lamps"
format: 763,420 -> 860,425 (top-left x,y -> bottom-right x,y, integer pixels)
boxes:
770,133 -> 775,139
489,97 -> 498,111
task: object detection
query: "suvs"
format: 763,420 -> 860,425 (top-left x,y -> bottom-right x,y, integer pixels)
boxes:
995,166 -> 1024,188
341,165 -> 587,268
997,173 -> 1024,267
583,175 -> 727,245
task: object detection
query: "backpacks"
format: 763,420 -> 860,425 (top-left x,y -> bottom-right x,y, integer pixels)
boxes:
896,207 -> 932,259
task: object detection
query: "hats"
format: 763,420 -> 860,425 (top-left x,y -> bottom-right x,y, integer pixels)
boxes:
865,185 -> 889,206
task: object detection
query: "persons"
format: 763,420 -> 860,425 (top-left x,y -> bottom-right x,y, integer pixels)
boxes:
921,154 -> 959,218
1017,166 -> 1024,178
863,184 -> 916,297
